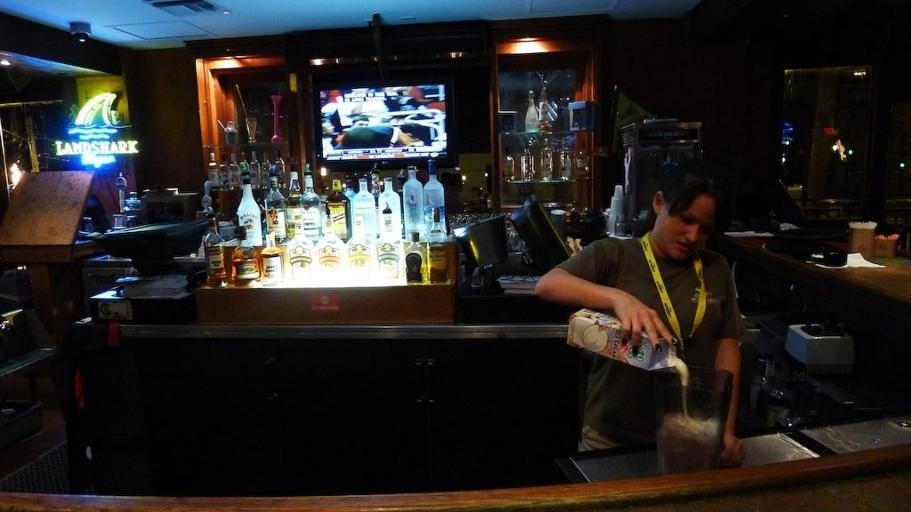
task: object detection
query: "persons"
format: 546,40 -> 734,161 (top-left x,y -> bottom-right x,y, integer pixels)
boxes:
333,123 -> 428,149
725,142 -> 806,234
532,168 -> 746,462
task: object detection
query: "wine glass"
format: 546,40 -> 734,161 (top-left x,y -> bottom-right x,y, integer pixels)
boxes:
247,117 -> 257,143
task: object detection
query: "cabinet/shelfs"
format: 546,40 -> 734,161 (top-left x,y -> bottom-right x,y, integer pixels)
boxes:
183,14 -> 613,231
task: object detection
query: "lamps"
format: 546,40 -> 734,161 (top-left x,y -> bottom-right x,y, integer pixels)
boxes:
496,37 -> 570,55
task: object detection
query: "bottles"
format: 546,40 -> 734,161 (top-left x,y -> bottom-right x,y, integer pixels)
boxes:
370,169 -> 383,233
286,172 -> 305,242
557,98 -> 564,129
746,360 -> 765,414
313,212 -> 348,287
567,308 -> 677,373
259,227 -> 282,290
405,166 -> 424,243
524,91 -> 538,133
299,169 -> 323,247
396,174 -> 406,235
427,208 -> 451,284
565,98 -> 573,129
283,215 -> 314,288
343,179 -> 357,200
791,378 -> 809,417
265,177 -> 287,245
208,154 -> 288,188
126,191 -> 143,228
405,230 -> 426,284
502,148 -> 514,177
540,140 -> 553,179
766,393 -> 787,425
379,179 -> 404,254
348,212 -> 376,287
327,179 -> 349,242
757,357 -> 779,417
237,174 -> 264,249
232,227 -> 260,289
522,143 -> 533,180
351,178 -> 377,249
558,139 -> 574,178
377,204 -> 403,287
539,81 -> 548,121
205,216 -> 229,287
423,159 -> 448,243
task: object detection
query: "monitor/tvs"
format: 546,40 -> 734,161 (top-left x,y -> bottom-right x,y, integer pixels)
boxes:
510,194 -> 571,273
312,77 -> 459,169
